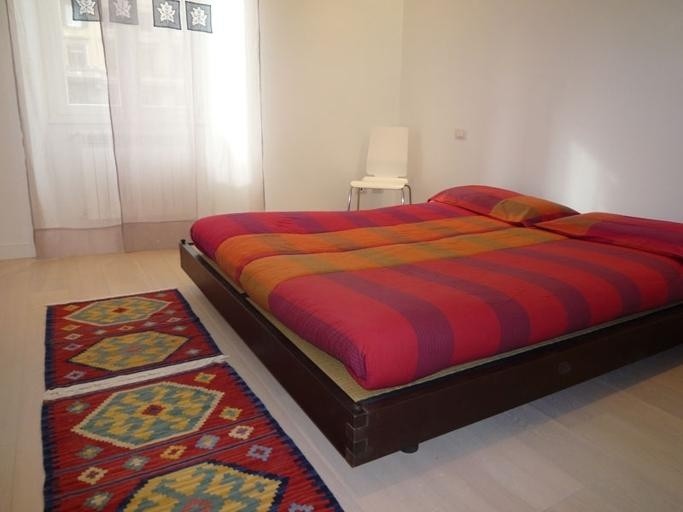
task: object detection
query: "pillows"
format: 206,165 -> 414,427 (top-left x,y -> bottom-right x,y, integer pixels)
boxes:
427,184 -> 580,229
534,212 -> 683,259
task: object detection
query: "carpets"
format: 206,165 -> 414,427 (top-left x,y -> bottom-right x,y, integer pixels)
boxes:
43,289 -> 341,512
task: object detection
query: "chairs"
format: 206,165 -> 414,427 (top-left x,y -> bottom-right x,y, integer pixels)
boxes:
347,126 -> 412,210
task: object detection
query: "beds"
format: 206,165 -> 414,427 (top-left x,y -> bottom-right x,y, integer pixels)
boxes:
179,202 -> 683,468
